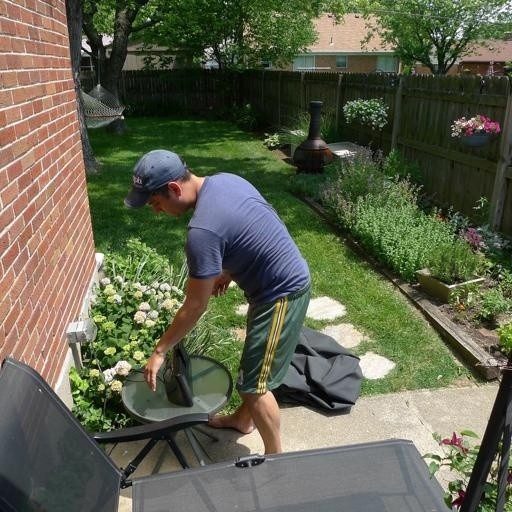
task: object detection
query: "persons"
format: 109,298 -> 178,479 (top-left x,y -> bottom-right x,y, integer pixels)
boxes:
121,148 -> 313,454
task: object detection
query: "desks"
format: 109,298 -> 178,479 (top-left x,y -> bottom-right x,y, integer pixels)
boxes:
117,355 -> 233,464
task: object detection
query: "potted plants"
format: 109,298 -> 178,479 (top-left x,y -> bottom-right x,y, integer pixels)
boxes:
289,103 -> 337,164
413,233 -> 490,301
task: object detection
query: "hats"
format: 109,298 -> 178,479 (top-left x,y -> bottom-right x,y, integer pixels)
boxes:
123,149 -> 187,209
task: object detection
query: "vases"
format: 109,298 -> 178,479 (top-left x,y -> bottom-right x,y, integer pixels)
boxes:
462,136 -> 489,147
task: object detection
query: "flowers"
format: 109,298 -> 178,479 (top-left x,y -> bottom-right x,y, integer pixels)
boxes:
449,111 -> 501,139
342,95 -> 393,135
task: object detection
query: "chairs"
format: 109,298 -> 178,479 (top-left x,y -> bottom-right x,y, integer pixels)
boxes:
1,358 -> 463,512
